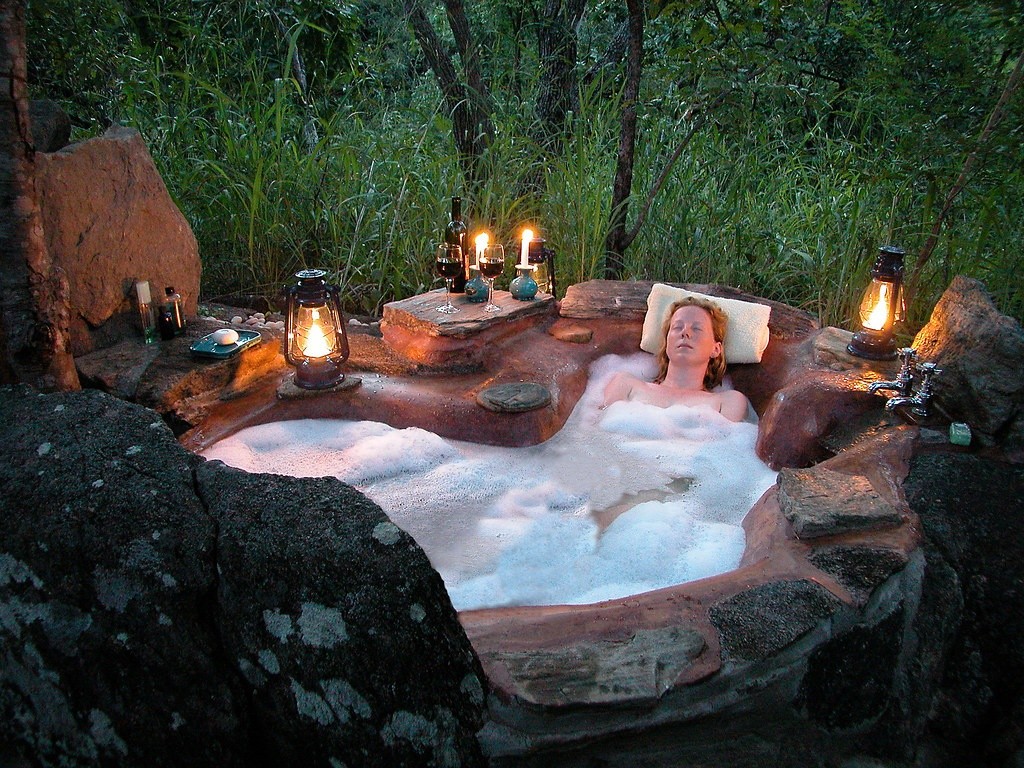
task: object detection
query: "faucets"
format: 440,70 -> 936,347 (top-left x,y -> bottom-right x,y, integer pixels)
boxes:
885,363 -> 942,417
868,348 -> 918,396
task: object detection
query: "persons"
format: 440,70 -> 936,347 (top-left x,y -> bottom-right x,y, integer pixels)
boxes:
603,297 -> 749,423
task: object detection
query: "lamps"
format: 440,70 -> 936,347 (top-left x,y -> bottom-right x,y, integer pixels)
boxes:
846,246 -> 904,362
284,268 -> 350,389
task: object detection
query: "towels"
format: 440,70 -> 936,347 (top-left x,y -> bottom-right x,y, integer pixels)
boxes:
640,283 -> 771,364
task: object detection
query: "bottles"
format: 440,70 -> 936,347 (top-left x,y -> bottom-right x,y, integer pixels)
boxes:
161,287 -> 187,335
445,196 -> 469,295
136,281 -> 156,344
160,312 -> 175,342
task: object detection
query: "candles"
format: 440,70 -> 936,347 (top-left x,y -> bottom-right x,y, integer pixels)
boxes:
520,228 -> 533,268
475,233 -> 489,268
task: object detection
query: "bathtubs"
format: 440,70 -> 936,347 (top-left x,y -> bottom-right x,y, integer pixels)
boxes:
75,279 -> 920,704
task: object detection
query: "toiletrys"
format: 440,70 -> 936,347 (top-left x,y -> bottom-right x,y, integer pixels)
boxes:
136,280 -> 183,344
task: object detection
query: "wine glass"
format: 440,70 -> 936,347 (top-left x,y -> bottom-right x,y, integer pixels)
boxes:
478,244 -> 505,313
436,244 -> 462,314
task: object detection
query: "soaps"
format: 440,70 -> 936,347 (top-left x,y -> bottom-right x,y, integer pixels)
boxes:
950,423 -> 971,447
213,329 -> 239,345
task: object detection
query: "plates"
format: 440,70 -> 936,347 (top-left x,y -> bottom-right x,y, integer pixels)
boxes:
189,328 -> 262,358
